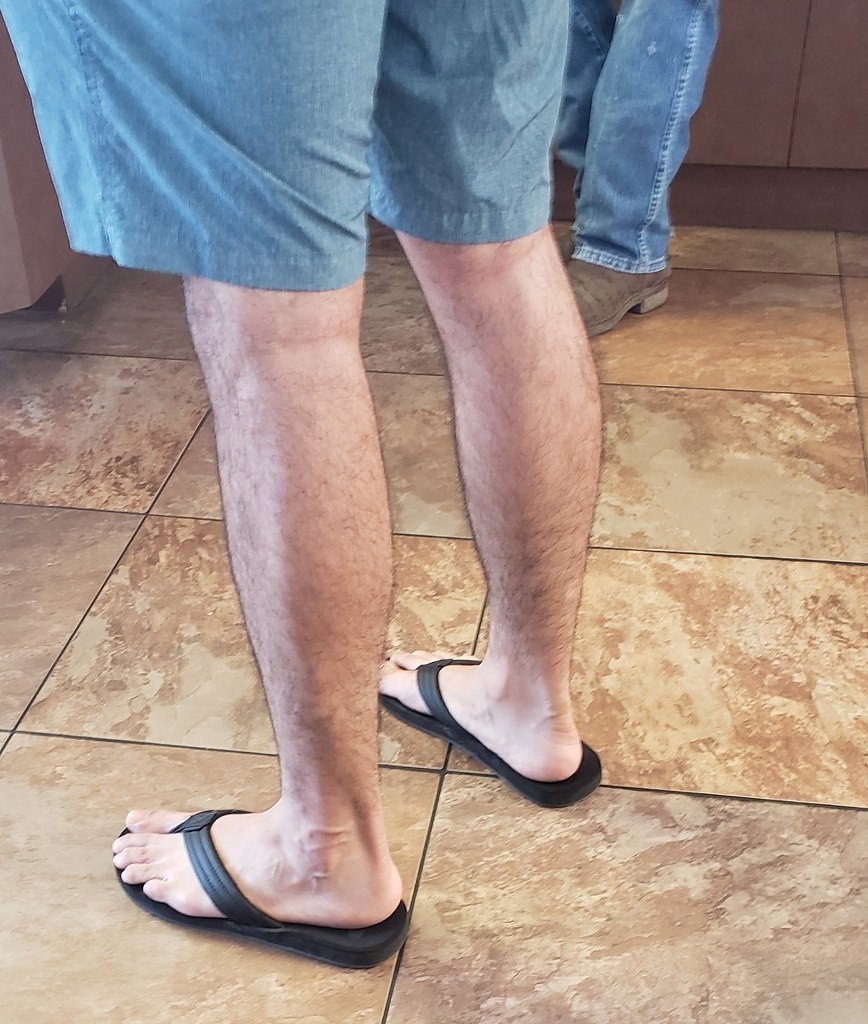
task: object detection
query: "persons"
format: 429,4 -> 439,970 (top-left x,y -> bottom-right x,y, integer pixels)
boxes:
0,0 -> 605,968
555,0 -> 720,337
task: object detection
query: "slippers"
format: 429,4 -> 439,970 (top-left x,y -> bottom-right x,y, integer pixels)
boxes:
375,657 -> 602,807
113,809 -> 409,969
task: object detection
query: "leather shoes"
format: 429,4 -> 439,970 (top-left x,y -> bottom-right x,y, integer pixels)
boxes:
565,258 -> 669,339
